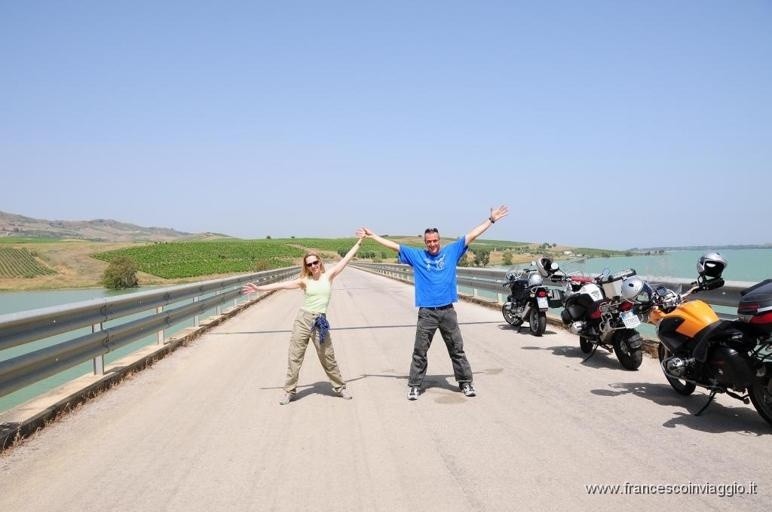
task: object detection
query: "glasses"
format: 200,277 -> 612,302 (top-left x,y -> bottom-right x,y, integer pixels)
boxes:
425,228 -> 437,233
306,261 -> 318,267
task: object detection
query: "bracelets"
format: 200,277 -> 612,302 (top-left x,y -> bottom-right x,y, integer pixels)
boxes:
490,218 -> 495,224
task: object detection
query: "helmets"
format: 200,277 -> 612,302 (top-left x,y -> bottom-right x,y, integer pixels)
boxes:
537,258 -> 553,276
621,276 -> 653,305
527,272 -> 543,287
697,251 -> 727,277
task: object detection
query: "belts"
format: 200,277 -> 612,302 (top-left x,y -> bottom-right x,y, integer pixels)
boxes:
429,303 -> 452,311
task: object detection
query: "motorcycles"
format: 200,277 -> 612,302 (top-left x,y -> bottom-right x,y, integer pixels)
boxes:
500,261 -> 644,369
634,276 -> 772,426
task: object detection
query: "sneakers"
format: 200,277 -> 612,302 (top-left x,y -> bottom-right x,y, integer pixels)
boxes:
337,389 -> 353,399
407,387 -> 419,400
279,392 -> 296,405
461,384 -> 476,396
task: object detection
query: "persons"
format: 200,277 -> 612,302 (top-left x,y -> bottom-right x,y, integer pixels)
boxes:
356,204 -> 509,400
242,232 -> 369,406
